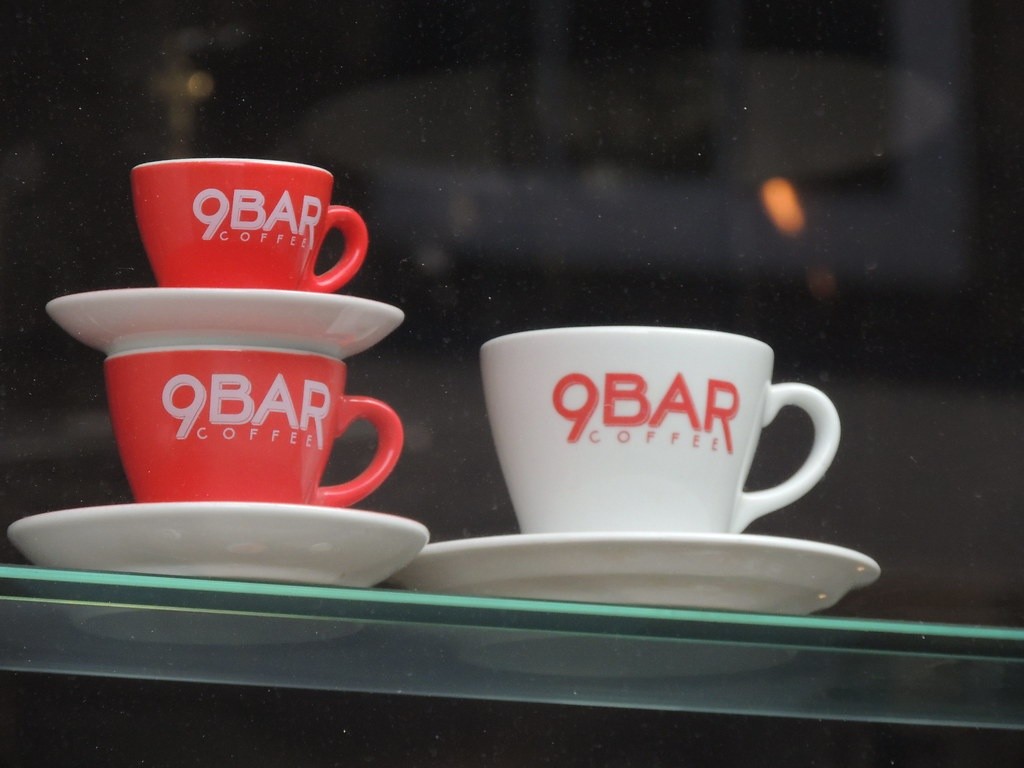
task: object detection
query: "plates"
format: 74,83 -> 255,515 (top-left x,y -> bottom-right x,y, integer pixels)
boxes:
385,529 -> 881,616
7,498 -> 429,590
44,288 -> 406,360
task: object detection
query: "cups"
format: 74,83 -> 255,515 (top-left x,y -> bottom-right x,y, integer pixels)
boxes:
131,158 -> 367,294
479,325 -> 843,538
101,345 -> 405,507
